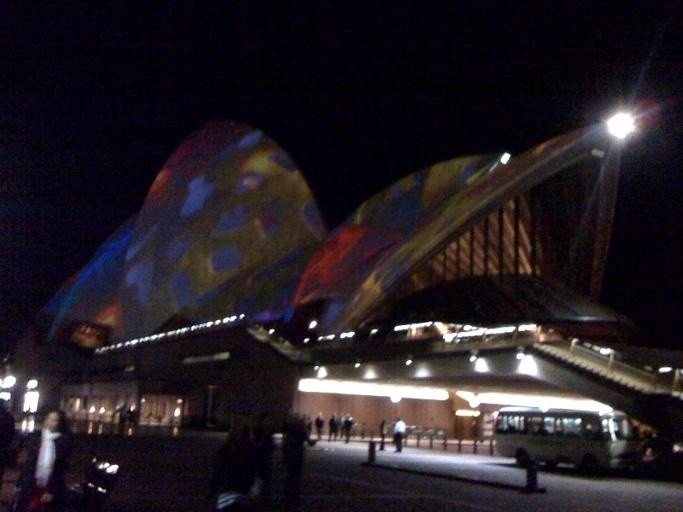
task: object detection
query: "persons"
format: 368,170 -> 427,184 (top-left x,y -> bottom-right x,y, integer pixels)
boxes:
278,413 -> 318,501
110,401 -> 133,435
393,416 -> 406,453
11,406 -> 66,510
203,420 -> 258,510
377,418 -> 389,451
342,412 -> 353,443
304,413 -> 312,437
315,411 -> 325,441
327,411 -> 339,441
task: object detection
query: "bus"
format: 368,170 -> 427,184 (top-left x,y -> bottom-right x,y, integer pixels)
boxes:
493,405 -> 637,472
